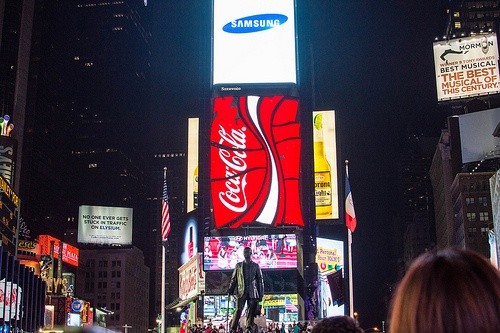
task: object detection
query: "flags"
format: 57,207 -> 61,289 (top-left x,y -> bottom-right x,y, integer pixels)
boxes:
344,168 -> 357,233
161,170 -> 171,240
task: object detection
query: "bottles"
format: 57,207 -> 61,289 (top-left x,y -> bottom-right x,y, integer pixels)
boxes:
314,114 -> 331,219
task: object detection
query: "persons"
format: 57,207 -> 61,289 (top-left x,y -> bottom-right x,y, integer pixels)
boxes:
226,247 -> 264,330
180,248 -> 378,333
388,247 -> 500,333
